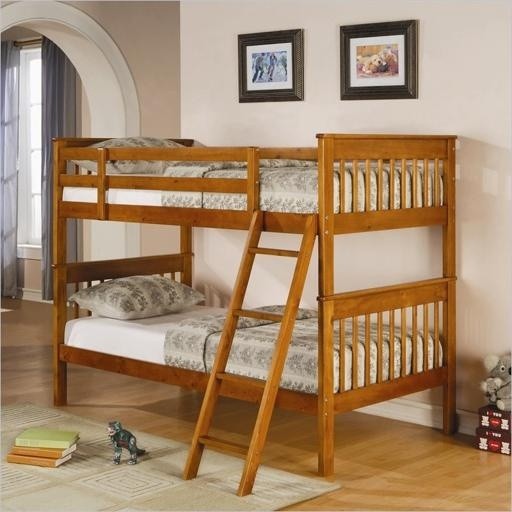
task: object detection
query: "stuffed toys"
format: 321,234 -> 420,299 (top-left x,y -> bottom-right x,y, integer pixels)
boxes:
477,352 -> 511,416
107,420 -> 146,465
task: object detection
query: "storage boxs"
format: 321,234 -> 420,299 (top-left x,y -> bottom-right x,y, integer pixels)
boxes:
475,427 -> 511,455
479,405 -> 511,431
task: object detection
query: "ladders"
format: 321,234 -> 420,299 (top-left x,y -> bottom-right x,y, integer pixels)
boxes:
182,209 -> 319,497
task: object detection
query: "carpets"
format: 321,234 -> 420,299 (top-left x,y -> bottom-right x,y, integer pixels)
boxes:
0,398 -> 340,512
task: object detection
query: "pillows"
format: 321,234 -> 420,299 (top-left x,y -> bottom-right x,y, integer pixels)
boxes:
70,135 -> 187,174
66,272 -> 207,319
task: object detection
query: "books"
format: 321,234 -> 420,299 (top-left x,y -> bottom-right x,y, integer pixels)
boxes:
6,425 -> 82,470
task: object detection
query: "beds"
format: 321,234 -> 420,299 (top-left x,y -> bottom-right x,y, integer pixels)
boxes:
50,131 -> 457,478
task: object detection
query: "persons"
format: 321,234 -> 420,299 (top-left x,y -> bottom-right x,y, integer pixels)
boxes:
252,53 -> 288,83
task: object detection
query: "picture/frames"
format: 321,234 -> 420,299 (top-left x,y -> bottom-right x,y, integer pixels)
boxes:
237,28 -> 304,103
340,20 -> 418,100
